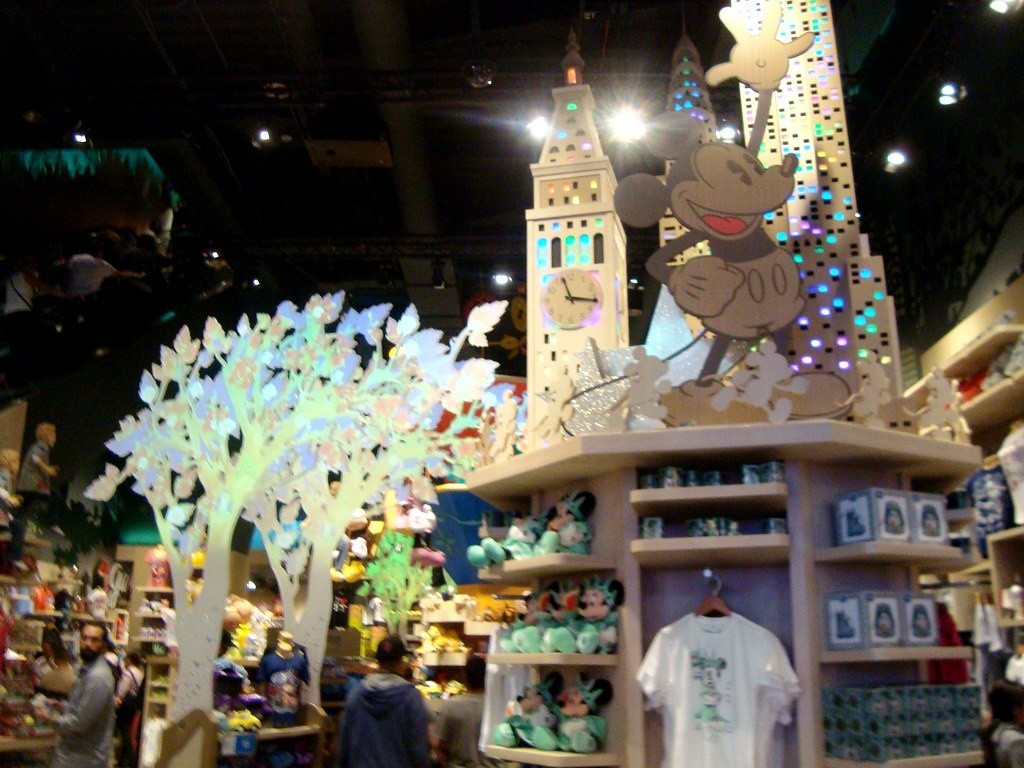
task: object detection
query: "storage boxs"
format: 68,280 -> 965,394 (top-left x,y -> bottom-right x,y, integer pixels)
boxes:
902,590 -> 939,645
826,590 -> 904,648
909,489 -> 951,546
823,683 -> 985,763
830,487 -> 912,545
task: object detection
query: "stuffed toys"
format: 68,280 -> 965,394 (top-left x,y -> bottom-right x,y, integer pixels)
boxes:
334,490 -> 627,753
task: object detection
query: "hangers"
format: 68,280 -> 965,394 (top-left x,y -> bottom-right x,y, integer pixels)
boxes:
694,575 -> 730,617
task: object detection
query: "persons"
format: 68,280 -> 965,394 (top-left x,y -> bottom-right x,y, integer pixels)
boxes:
33,621 -> 239,760
35,620 -> 115,768
979,680 -> 1024,768
257,632 -> 309,726
145,545 -> 205,585
0,424 -> 56,571
339,635 -> 432,768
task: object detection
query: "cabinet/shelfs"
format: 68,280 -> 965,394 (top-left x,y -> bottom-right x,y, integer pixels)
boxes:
465,420 -> 989,768
903,277 -> 1024,629
0,400 -> 510,768
